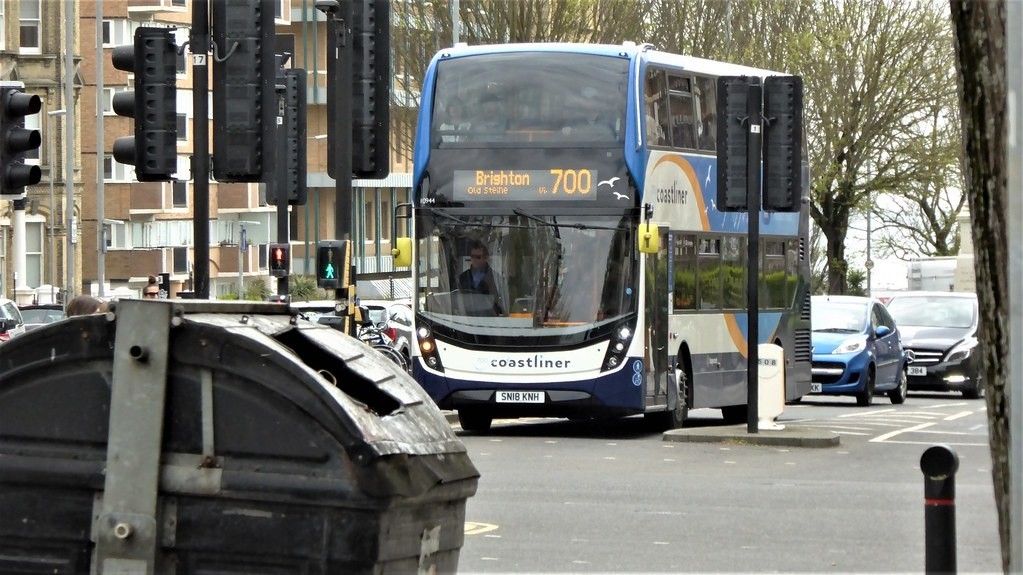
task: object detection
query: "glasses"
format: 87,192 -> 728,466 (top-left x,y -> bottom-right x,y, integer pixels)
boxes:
471,254 -> 481,259
146,292 -> 158,297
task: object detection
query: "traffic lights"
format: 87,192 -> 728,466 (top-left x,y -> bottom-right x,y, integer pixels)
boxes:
110,24 -> 177,184
270,244 -> 289,277
1,81 -> 41,200
316,240 -> 351,288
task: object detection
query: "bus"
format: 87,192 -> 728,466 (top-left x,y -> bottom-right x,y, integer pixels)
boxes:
390,38 -> 813,435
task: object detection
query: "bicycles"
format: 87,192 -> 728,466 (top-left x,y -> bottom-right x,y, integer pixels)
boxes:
358,321 -> 410,374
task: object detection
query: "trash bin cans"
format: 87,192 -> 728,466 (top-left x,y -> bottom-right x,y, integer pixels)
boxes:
0,290 -> 481,575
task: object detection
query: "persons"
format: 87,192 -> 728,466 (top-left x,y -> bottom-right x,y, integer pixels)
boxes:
67,296 -> 117,317
561,86 -> 616,136
439,95 -> 471,143
142,273 -> 159,299
471,92 -> 514,142
457,240 -> 506,317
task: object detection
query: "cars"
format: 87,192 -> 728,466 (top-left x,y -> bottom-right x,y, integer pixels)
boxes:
882,289 -> 984,399
789,294 -> 910,406
290,299 -> 412,369
